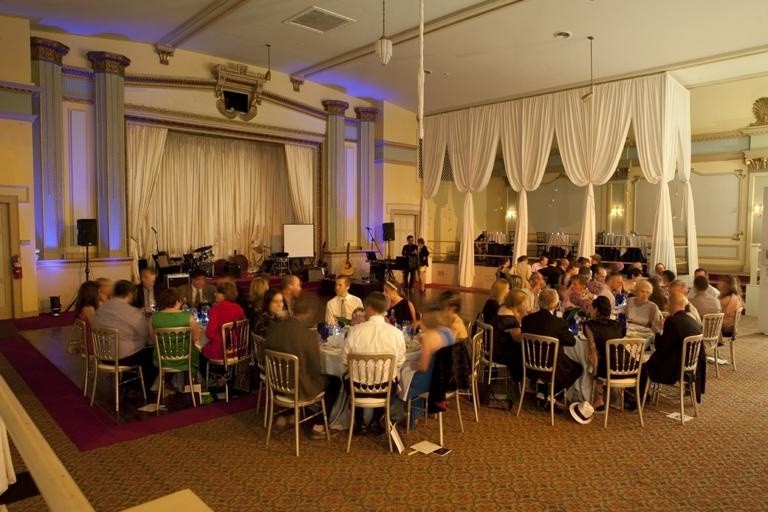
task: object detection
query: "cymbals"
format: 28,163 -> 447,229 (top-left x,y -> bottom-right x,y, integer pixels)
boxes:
193,245 -> 212,251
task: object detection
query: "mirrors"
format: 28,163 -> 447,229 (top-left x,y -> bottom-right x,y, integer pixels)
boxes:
746,172 -> 768,269
606,180 -> 635,234
506,187 -> 519,237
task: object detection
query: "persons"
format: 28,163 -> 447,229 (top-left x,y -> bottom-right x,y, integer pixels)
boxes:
402,235 -> 429,293
75,269 -> 269,397
473,255 -> 743,412
259,276 -> 471,441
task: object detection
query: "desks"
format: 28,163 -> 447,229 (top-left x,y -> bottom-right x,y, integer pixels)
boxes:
164,273 -> 191,289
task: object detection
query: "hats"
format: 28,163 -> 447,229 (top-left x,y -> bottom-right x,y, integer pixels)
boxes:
569,401 -> 594,425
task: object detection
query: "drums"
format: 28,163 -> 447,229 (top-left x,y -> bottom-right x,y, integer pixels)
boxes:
200,249 -> 214,262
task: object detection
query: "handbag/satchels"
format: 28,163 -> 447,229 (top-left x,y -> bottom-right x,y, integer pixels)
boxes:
66,326 -> 80,354
328,392 -> 350,430
470,383 -> 492,405
233,372 -> 250,392
248,361 -> 260,390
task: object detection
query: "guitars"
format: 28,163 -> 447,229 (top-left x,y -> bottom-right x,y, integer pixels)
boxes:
341,243 -> 354,276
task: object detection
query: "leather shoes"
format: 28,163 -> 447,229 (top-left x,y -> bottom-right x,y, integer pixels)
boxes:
275,412 -> 418,440
536,395 -> 652,414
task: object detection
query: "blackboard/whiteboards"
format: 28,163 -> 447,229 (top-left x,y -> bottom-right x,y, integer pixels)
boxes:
283,224 -> 315,258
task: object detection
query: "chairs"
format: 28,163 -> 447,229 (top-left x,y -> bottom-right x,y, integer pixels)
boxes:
537,232 -> 546,255
509,231 -> 515,252
475,306 -> 746,430
74,318 -> 252,416
250,310 -> 485,455
572,233 -> 579,256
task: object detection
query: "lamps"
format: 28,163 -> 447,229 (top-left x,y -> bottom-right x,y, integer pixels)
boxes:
265,43 -> 273,80
375,0 -> 394,65
581,36 -> 594,102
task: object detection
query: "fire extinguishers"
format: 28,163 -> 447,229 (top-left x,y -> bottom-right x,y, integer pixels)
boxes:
11,254 -> 22,279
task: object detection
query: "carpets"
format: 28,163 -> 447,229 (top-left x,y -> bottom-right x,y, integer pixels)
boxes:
0,284 -> 489,454
